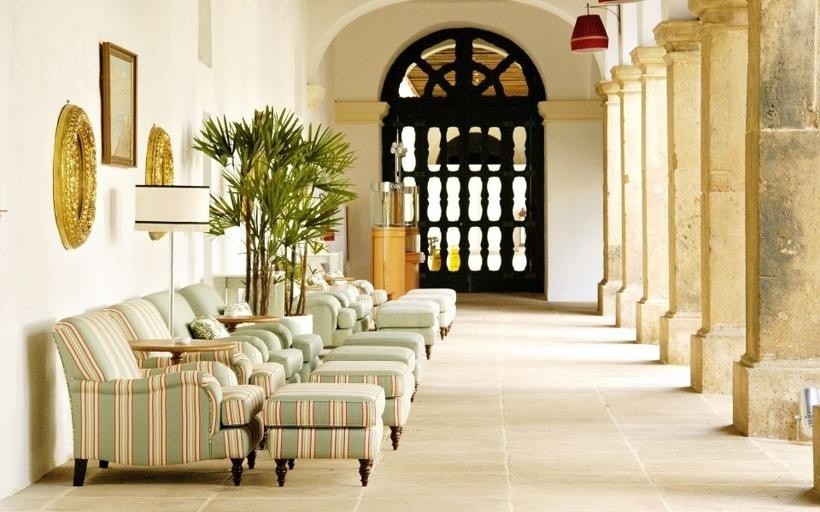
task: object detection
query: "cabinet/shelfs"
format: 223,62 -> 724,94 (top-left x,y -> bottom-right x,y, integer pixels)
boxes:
371,224 -> 422,301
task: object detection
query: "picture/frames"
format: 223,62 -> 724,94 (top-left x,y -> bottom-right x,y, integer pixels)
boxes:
144,124 -> 174,241
99,42 -> 139,168
53,106 -> 97,250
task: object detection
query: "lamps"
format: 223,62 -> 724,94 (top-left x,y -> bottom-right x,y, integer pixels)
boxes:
132,184 -> 212,338
570,2 -> 621,52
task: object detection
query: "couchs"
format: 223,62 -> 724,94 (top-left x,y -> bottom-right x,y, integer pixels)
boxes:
51,308 -> 264,485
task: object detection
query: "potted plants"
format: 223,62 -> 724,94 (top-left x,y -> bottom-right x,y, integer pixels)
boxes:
280,128 -> 357,335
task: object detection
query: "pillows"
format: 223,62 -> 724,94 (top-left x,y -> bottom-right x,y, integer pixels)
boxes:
190,314 -> 228,340
223,301 -> 256,326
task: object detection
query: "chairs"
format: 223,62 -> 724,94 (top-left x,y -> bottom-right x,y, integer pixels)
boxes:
111,297 -> 285,450
329,266 -> 388,304
182,282 -> 323,383
309,272 -> 373,332
144,291 -> 304,385
303,288 -> 356,347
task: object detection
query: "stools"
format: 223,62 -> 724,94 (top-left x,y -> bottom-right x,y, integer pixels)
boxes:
402,288 -> 458,338
310,361 -> 414,450
377,300 -> 440,359
263,382 -> 386,487
325,342 -> 417,401
343,330 -> 427,382
